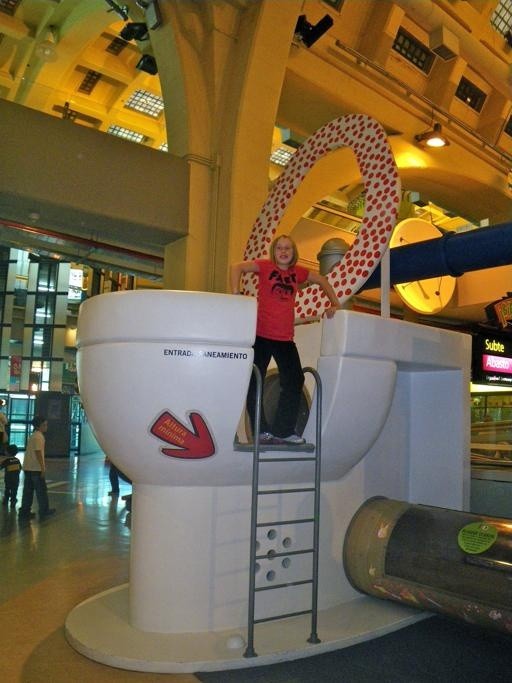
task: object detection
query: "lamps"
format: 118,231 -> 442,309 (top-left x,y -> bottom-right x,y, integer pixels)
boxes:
415,123 -> 450,146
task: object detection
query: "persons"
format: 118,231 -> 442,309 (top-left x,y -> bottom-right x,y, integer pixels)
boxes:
0,444 -> 22,510
0,400 -> 9,455
226,233 -> 342,446
107,462 -> 132,496
17,414 -> 57,517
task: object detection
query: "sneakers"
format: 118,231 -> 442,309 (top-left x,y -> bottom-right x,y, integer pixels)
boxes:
19,512 -> 35,520
123,494 -> 132,499
41,508 -> 56,517
281,434 -> 306,445
254,433 -> 285,445
109,491 -> 119,495
